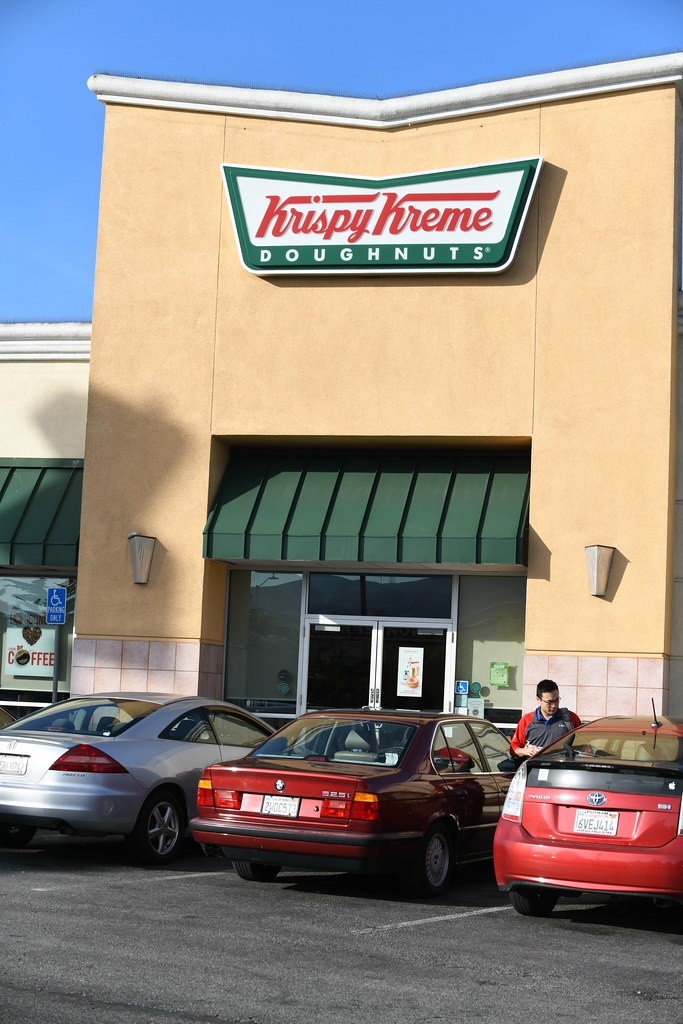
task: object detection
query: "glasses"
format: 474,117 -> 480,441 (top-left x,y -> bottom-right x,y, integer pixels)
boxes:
539,697 -> 561,705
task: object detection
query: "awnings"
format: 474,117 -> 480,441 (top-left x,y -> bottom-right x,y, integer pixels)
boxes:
0,458 -> 85,569
201,453 -> 529,566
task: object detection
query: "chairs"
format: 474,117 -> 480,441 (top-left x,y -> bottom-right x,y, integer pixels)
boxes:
96,716 -> 121,731
52,719 -> 75,730
635,743 -> 669,760
334,727 -> 380,761
173,720 -> 201,741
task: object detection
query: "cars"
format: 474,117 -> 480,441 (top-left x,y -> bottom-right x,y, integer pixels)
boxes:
493,698 -> 683,917
0,692 -> 277,865
189,709 -> 527,897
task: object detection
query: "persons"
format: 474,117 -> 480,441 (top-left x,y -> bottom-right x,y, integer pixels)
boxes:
433,747 -> 475,774
510,680 -> 592,756
